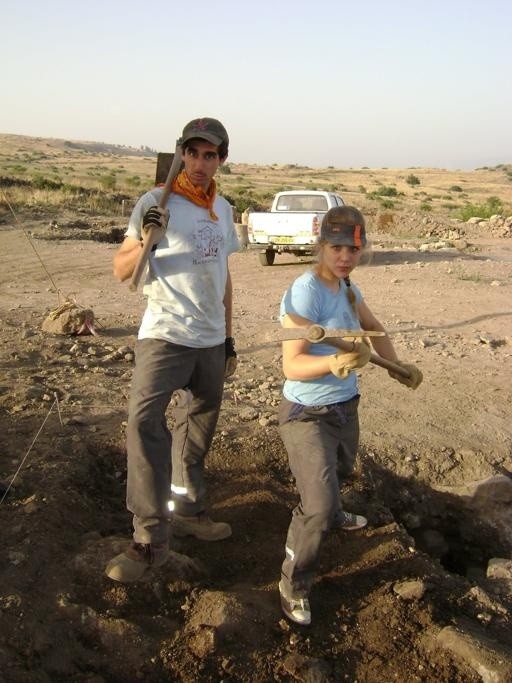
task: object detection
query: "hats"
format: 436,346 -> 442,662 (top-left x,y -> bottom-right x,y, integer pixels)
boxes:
180,116 -> 230,149
320,204 -> 368,249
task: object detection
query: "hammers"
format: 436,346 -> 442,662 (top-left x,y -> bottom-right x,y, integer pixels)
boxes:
262,324 -> 412,378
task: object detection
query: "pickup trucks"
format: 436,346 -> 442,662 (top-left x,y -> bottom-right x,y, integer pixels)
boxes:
247,190 -> 346,266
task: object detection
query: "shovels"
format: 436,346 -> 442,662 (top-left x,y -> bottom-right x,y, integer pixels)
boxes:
129,139 -> 185,293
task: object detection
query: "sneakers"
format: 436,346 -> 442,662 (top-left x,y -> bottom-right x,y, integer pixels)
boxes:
105,536 -> 172,584
171,510 -> 233,544
278,581 -> 311,626
331,510 -> 368,532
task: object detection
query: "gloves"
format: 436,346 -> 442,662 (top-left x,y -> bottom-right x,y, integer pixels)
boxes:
138,205 -> 171,253
328,334 -> 372,381
224,336 -> 239,380
387,359 -> 424,390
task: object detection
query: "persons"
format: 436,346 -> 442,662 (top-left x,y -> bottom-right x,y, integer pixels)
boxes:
279,206 -> 423,626
105,118 -> 240,583
